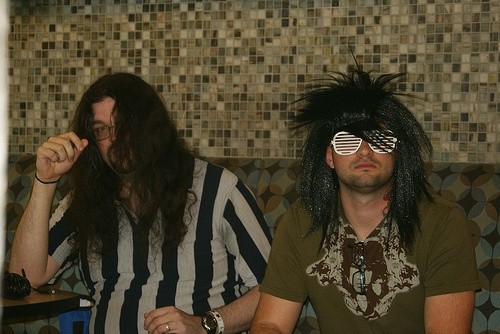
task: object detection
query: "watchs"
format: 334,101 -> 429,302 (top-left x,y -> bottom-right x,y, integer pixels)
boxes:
201,313 -> 217,334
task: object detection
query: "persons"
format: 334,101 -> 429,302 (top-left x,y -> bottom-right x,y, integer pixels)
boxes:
8,73 -> 274,334
250,45 -> 480,334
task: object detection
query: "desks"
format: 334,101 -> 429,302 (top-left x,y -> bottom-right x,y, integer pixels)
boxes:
0,287 -> 80,324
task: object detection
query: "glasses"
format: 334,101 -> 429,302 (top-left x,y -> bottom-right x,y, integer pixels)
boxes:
89,124 -> 119,141
331,130 -> 397,155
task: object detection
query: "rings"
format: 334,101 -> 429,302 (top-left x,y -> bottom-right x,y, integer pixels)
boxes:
165,323 -> 170,332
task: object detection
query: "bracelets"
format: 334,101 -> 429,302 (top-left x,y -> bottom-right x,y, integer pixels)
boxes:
35,174 -> 60,184
208,310 -> 224,334
